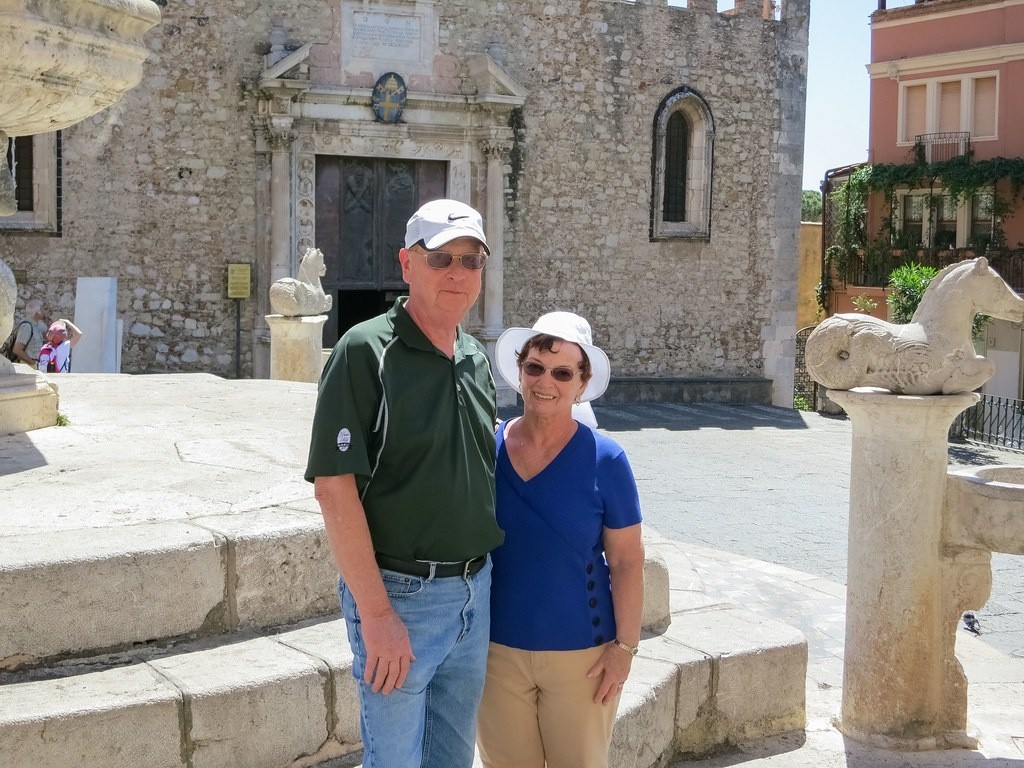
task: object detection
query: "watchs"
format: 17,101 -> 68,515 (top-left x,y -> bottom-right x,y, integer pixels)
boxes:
615,639 -> 638,656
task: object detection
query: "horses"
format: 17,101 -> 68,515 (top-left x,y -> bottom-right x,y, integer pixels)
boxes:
269,247 -> 332,317
804,256 -> 1024,396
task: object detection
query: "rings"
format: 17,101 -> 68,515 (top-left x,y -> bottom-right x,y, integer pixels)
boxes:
619,684 -> 623,687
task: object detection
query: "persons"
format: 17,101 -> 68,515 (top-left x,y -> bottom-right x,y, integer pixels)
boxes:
476,311 -> 645,768
302,198 -> 506,768
13,303 -> 44,370
37,319 -> 82,373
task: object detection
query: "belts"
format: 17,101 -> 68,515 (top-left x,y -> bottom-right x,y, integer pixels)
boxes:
375,553 -> 487,578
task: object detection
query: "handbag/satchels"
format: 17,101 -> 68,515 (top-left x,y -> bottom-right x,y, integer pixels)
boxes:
3,321 -> 33,363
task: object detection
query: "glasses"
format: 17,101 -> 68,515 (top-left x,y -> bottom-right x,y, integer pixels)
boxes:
410,249 -> 487,270
521,361 -> 584,382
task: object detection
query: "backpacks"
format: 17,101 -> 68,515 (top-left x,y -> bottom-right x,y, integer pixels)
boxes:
36,341 -> 66,373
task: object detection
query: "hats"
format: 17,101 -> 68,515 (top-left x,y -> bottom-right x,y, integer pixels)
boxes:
404,199 -> 491,256
495,311 -> 610,404
